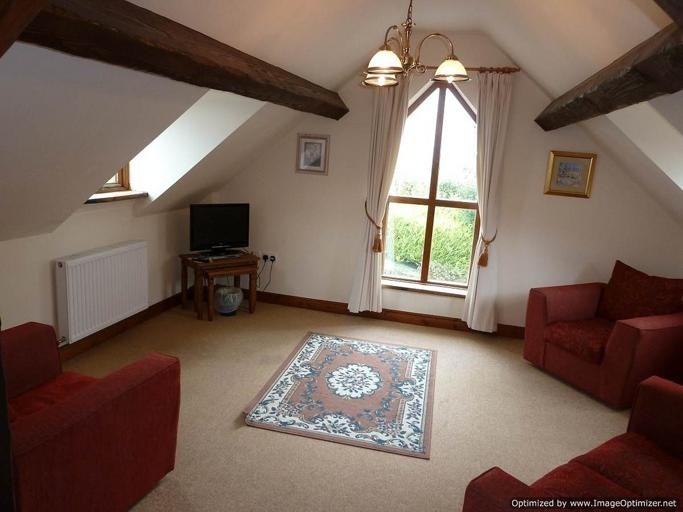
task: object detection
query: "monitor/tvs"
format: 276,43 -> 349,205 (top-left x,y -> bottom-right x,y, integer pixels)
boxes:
188,203 -> 251,259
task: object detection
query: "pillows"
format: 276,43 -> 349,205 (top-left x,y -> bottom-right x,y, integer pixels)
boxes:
597,260 -> 683,322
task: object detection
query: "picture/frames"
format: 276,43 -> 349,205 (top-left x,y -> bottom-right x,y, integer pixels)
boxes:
544,150 -> 598,199
295,133 -> 330,176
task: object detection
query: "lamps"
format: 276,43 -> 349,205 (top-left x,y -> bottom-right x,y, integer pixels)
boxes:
361,0 -> 469,87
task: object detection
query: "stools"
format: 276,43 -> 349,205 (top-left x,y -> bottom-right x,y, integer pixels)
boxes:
204,264 -> 259,322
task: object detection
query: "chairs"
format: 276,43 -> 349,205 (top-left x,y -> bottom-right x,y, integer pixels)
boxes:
463,376 -> 683,512
0,321 -> 181,512
523,281 -> 683,410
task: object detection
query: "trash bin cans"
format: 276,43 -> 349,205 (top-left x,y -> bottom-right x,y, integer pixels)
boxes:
216,287 -> 241,316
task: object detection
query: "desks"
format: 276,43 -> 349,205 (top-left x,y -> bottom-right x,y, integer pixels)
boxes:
179,248 -> 257,314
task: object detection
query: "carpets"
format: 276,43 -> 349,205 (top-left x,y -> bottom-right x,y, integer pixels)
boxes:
240,331 -> 437,461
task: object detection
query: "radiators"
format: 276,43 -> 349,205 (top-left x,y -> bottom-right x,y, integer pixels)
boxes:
53,240 -> 149,347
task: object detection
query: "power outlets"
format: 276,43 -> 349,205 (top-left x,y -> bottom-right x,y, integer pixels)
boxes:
262,253 -> 278,264
